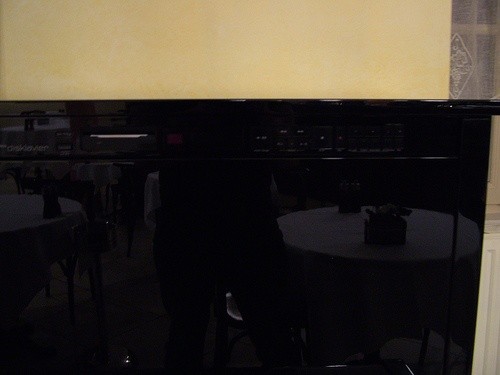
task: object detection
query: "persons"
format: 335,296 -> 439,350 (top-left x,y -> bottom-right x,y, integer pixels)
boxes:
230,165 -> 329,370
152,99 -> 324,375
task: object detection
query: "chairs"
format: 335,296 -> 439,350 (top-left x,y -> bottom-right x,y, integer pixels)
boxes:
211,298 -> 262,368
9,174 -> 148,263
276,177 -> 306,215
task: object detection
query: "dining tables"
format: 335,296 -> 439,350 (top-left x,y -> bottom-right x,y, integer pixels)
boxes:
274,204 -> 484,375
0,194 -> 107,374
144,169 -> 278,231
0,125 -> 153,223
